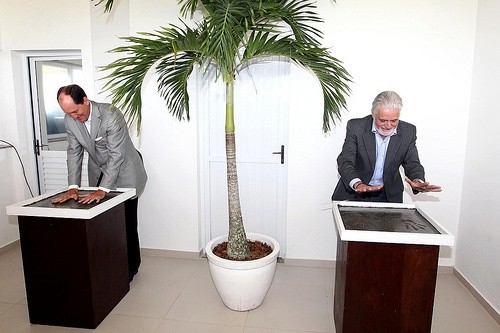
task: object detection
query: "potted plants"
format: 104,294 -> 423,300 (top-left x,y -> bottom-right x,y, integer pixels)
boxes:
96,0 -> 353,312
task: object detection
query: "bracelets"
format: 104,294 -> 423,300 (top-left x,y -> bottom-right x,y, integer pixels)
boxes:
356,183 -> 364,189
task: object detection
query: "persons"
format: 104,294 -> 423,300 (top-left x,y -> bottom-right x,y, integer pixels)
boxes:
331,91 -> 442,204
51,85 -> 148,283
